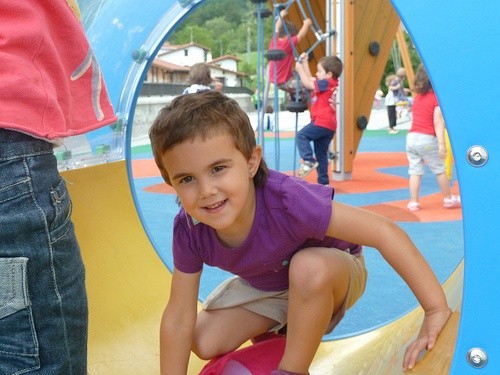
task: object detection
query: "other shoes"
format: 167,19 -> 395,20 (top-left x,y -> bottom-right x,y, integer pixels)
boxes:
388,129 -> 399,135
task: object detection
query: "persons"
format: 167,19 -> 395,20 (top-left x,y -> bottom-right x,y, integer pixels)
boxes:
373,68 -> 407,134
181,62 -> 223,95
148,89 -> 453,375
0,0 -> 119,375
267,9 -> 313,110
406,62 -> 462,212
294,52 -> 343,187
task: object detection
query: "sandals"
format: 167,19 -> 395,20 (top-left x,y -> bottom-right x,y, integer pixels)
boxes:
406,202 -> 420,212
443,194 -> 462,208
297,160 -> 320,178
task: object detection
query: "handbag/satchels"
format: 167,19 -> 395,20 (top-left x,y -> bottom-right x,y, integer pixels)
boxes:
384,87 -> 397,107
